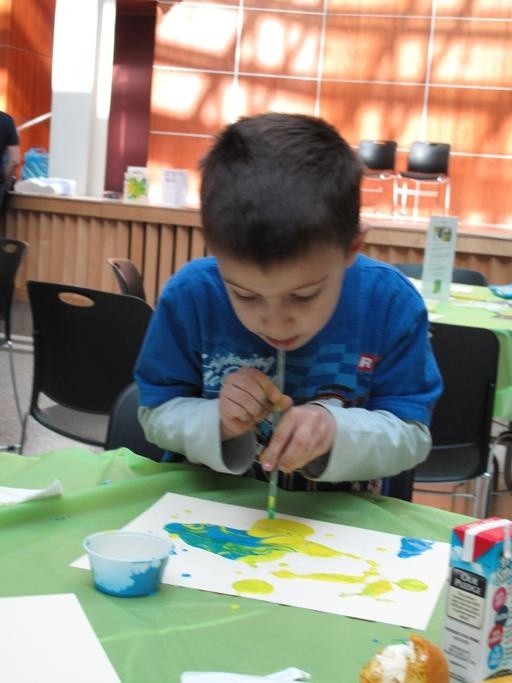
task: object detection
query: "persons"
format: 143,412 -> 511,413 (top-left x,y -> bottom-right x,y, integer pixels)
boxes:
131,114 -> 445,498
0,110 -> 20,248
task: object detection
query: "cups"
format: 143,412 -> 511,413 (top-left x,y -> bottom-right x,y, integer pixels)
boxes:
84,529 -> 175,599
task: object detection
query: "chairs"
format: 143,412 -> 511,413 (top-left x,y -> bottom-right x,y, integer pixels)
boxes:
393,263 -> 487,287
108,257 -> 147,303
357,139 -> 398,215
0,238 -> 29,451
400,141 -> 451,217
106,382 -> 413,501
19,280 -> 154,456
413,321 -> 500,518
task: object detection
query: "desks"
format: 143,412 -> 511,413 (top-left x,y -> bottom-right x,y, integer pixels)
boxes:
0,447 -> 512,683
406,276 -> 512,498
